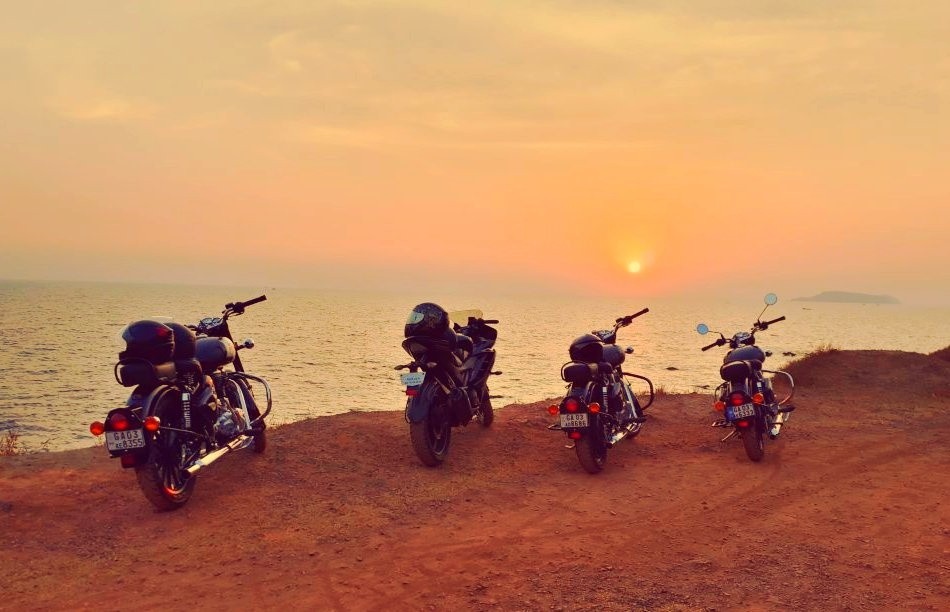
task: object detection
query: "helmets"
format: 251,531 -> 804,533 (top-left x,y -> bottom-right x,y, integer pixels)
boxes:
115,319 -> 175,365
403,301 -> 451,340
569,333 -> 605,364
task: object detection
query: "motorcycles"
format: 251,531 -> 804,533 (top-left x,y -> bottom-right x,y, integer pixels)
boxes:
90,294 -> 272,512
694,292 -> 797,462
392,302 -> 505,468
547,306 -> 655,475
